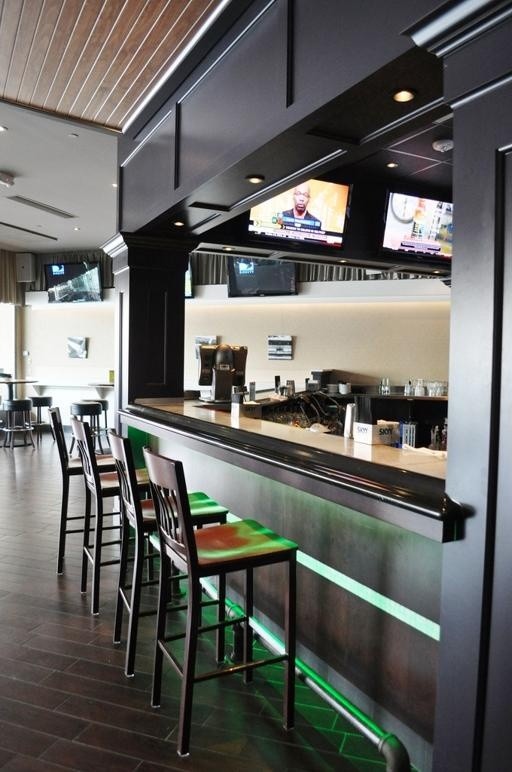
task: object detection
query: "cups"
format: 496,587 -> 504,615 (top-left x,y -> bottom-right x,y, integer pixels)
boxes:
284,385 -> 294,396
403,377 -> 448,397
342,403 -> 358,438
378,376 -> 391,395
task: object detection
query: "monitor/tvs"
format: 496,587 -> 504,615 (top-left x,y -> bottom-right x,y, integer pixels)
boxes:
244,176 -> 354,252
376,187 -> 453,265
184,255 -> 194,298
44,261 -> 103,303
228,256 -> 298,298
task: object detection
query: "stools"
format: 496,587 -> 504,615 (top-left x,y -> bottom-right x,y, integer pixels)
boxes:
81,399 -> 110,449
69,402 -> 104,457
25,397 -> 55,440
2,400 -> 35,450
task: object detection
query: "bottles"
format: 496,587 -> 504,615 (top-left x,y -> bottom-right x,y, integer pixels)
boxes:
430,424 -> 447,450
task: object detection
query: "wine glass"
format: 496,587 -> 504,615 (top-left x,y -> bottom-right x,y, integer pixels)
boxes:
263,403 -> 306,427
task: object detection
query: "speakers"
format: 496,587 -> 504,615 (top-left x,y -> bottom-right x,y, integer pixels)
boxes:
15,253 -> 36,282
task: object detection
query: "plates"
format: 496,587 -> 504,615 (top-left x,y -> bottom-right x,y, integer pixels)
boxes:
326,382 -> 352,395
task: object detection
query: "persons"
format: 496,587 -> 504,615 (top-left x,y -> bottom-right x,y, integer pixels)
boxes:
279,182 -> 320,235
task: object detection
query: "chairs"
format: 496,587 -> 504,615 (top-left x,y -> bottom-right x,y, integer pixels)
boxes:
143,445 -> 298,758
50,407 -> 122,575
106,428 -> 230,677
71,415 -> 181,616
